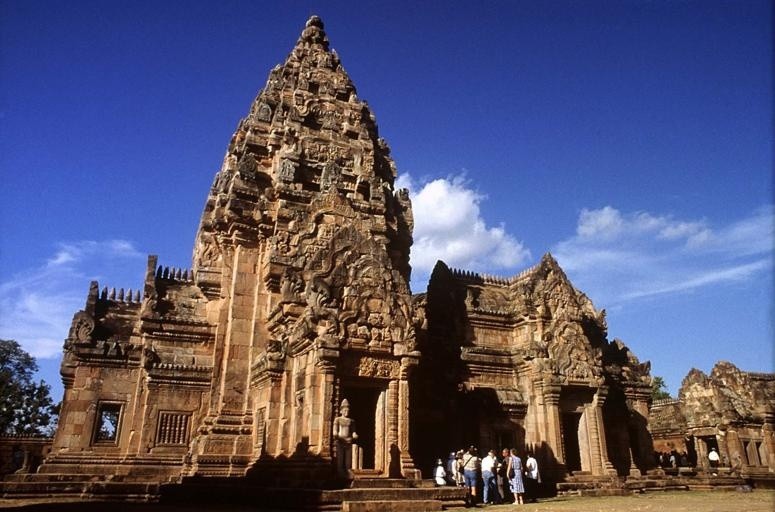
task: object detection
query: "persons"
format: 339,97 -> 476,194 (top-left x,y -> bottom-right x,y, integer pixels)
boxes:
431,442 -> 544,506
707,447 -> 721,468
330,397 -> 360,482
653,451 -> 693,469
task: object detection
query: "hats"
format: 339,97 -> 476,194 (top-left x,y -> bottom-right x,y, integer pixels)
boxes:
456,451 -> 463,458
435,458 -> 443,465
448,452 -> 457,459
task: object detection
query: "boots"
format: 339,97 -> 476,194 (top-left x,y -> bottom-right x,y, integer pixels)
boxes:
465,495 -> 482,509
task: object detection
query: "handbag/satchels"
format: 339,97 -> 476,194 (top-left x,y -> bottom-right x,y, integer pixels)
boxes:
509,468 -> 515,480
537,470 -> 542,484
459,465 -> 464,474
499,461 -> 507,477
